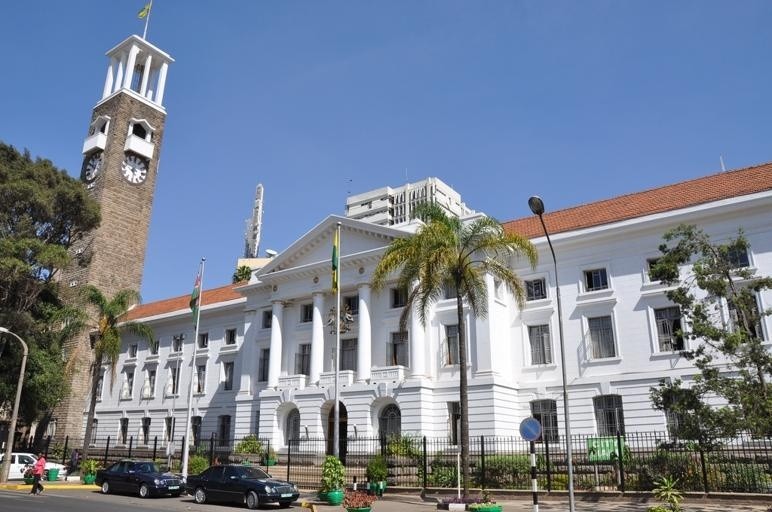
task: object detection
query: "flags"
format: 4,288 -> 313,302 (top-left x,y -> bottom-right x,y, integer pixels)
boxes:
188,257 -> 204,332
330,221 -> 341,296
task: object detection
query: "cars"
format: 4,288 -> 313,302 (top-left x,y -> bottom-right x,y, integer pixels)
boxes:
0,452 -> 70,481
96,460 -> 185,497
186,465 -> 300,509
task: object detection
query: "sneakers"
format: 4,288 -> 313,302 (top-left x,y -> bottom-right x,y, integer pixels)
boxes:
29,493 -> 37,496
39,489 -> 45,495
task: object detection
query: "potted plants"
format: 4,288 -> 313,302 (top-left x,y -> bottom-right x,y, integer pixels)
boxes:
319,454 -> 347,506
318,479 -> 328,501
20,462 -> 35,485
366,453 -> 388,496
342,489 -> 377,512
47,441 -> 65,481
230,433 -> 265,466
467,489 -> 502,512
79,458 -> 99,484
261,444 -> 277,466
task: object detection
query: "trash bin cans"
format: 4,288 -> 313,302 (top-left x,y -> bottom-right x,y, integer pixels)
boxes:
49,469 -> 57,481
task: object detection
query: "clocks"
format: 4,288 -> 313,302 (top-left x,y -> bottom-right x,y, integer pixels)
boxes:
120,153 -> 148,186
84,149 -> 103,183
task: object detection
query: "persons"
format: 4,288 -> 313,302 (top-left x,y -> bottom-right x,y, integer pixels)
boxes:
29,450 -> 46,498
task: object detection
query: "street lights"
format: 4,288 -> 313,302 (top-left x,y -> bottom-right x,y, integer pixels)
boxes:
528,194 -> 577,512
0,326 -> 29,483
